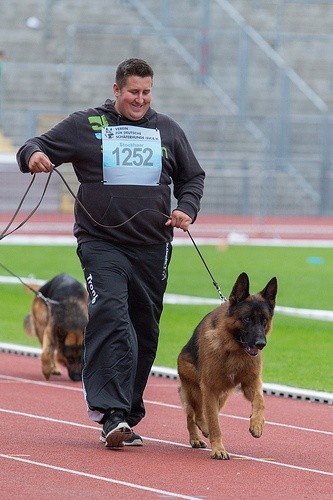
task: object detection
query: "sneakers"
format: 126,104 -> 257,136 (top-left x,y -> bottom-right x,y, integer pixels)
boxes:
99,415 -> 143,450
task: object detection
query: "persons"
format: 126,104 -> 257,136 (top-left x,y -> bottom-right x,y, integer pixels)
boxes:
16,58 -> 205,447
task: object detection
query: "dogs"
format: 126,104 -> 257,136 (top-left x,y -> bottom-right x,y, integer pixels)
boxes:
32,272 -> 89,382
178,272 -> 277,459
